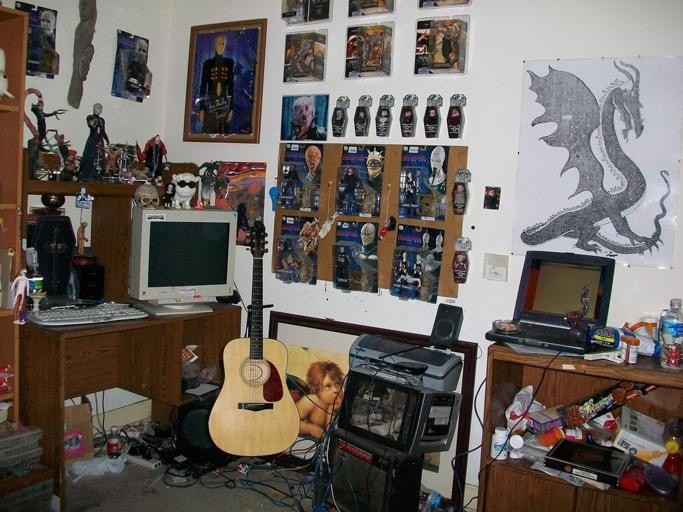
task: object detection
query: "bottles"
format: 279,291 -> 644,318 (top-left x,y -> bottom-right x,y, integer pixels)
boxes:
663,416 -> 682,454
626,338 -> 639,366
510,434 -> 525,449
490,426 -> 508,460
621,335 -> 632,360
107,427 -> 123,459
658,298 -> 683,370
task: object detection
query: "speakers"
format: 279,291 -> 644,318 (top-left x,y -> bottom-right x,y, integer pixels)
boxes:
430,303 -> 463,348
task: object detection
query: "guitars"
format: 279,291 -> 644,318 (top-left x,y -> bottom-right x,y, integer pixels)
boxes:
207,218 -> 300,458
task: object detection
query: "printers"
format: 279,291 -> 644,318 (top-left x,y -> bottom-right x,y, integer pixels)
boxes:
349,334 -> 462,392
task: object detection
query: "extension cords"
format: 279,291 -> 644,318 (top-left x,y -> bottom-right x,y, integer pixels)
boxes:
122,450 -> 161,470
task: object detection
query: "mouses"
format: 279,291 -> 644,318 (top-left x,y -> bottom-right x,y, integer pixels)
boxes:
398,362 -> 428,375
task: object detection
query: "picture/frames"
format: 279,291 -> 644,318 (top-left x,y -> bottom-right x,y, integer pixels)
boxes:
268,310 -> 478,512
183,18 -> 267,144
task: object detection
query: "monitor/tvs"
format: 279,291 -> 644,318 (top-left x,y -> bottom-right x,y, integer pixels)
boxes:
321,429 -> 423,512
130,206 -> 238,315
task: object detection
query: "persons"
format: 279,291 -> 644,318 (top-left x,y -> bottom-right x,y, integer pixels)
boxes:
76,188 -> 88,202
128,39 -> 152,101
31,101 -> 67,142
12,268 -> 30,310
79,104 -> 110,181
297,360 -> 345,438
41,11 -> 54,34
487,190 -> 496,209
163,184 -> 175,207
198,35 -> 236,134
148,135 -> 167,175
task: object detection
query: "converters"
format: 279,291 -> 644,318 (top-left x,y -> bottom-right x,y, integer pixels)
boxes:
171,454 -> 190,471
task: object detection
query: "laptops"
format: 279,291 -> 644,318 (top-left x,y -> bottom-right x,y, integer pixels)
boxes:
485,251 -> 615,355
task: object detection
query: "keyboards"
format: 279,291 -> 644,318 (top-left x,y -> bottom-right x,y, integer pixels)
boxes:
26,303 -> 149,326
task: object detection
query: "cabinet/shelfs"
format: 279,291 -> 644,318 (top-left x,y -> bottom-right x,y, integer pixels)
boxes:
478,341 -> 683,512
0,5 -> 30,426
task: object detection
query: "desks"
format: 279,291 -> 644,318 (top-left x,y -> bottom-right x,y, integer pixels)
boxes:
20,148 -> 242,512
